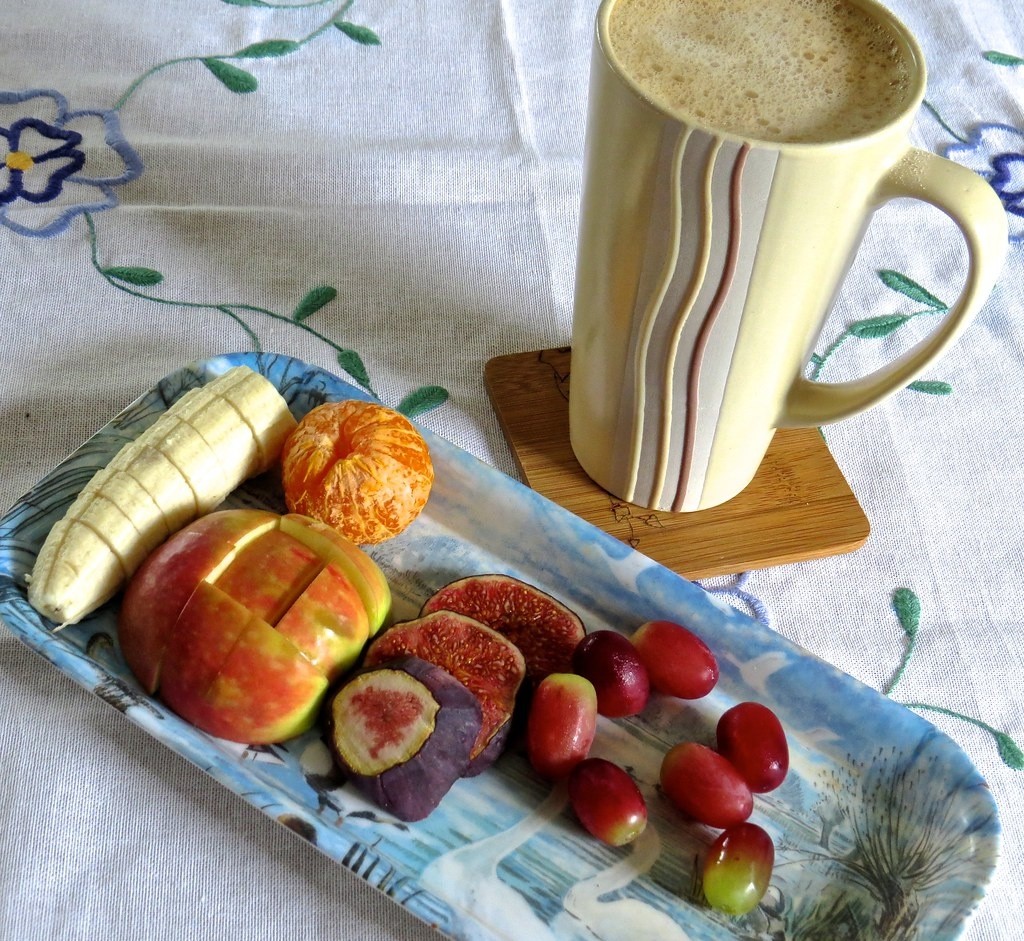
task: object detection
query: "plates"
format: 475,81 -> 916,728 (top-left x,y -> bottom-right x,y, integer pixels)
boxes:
0,351 -> 999,941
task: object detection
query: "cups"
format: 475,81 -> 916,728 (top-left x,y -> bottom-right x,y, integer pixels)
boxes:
568,0 -> 1009,512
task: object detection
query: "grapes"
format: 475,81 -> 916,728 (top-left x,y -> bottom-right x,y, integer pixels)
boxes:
524,621 -> 790,917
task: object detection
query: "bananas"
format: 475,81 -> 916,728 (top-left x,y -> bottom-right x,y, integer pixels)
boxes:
28,366 -> 301,626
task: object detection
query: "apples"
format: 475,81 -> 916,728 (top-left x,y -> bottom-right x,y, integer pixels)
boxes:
118,509 -> 394,744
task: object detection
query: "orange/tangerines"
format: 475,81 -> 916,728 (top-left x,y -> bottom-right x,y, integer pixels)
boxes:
281,399 -> 434,545
